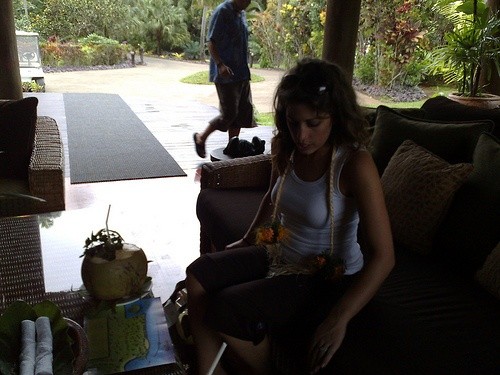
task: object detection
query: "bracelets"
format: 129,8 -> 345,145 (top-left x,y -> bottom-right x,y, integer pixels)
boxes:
242,238 -> 250,246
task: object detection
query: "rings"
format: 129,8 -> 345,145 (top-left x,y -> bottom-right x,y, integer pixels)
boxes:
320,347 -> 327,352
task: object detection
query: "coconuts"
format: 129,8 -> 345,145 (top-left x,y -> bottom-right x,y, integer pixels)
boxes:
81,242 -> 148,300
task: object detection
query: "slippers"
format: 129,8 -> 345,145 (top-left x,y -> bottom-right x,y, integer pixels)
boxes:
193,133 -> 207,158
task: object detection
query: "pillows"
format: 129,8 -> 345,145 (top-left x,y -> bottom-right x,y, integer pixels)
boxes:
357,104 -> 500,298
0,96 -> 38,184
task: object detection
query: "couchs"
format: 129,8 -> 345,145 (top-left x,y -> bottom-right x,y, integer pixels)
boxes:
197,96 -> 500,375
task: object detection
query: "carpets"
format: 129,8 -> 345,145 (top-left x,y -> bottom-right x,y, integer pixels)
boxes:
63,92 -> 187,184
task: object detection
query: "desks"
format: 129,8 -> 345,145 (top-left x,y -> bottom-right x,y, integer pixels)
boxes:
0,288 -> 191,375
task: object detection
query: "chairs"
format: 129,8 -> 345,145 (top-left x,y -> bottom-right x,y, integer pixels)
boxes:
0,100 -> 65,218
0,215 -> 45,296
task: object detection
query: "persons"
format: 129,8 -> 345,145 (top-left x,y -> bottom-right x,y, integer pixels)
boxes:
185,60 -> 395,375
193,0 -> 258,159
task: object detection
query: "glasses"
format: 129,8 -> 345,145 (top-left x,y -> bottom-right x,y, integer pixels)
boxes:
282,74 -> 327,95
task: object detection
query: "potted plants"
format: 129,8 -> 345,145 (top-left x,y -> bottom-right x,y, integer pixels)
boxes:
446,7 -> 500,111
